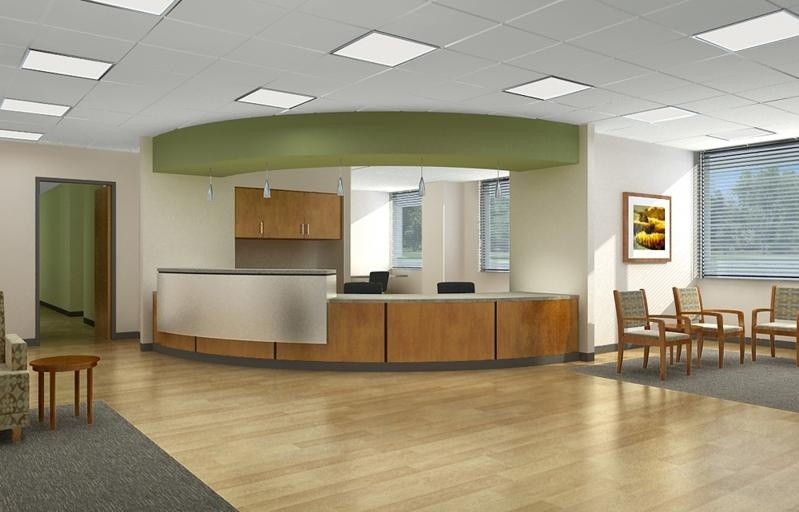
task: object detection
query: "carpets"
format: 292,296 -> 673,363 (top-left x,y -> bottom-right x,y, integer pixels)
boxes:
0,400 -> 241,512
564,346 -> 799,413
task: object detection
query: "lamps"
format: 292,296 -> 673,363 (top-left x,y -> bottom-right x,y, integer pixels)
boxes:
205,158 -> 503,203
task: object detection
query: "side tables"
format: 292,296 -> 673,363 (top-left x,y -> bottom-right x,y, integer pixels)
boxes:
29,355 -> 102,431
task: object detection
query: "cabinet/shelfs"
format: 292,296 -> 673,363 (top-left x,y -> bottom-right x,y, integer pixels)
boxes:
235,187 -> 267,240
267,189 -> 342,241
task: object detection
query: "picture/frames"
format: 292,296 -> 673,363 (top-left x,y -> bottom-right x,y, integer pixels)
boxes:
622,192 -> 673,265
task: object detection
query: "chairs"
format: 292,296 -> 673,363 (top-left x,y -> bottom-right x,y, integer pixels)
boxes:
611,283 -> 799,380
0,289 -> 30,443
343,270 -> 390,294
436,280 -> 477,295
